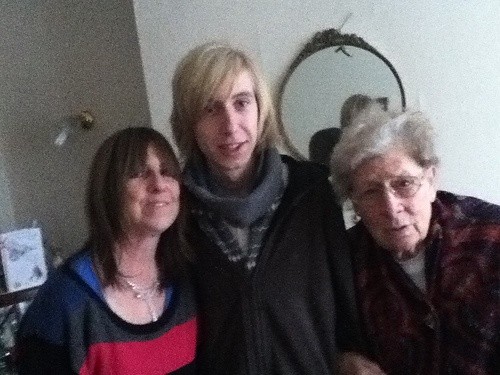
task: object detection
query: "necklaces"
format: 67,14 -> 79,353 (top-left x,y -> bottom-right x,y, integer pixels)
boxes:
115,273 -> 162,323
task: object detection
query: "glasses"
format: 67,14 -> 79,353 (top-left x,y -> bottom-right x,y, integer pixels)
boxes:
350,167 -> 430,206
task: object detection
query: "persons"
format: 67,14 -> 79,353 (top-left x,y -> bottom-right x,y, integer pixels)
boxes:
11,127 -> 201,375
340,94 -> 374,127
309,127 -> 344,165
165,42 -> 360,375
329,107 -> 500,375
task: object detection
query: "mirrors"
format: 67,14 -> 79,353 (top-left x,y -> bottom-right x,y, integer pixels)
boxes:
274,28 -> 407,173
